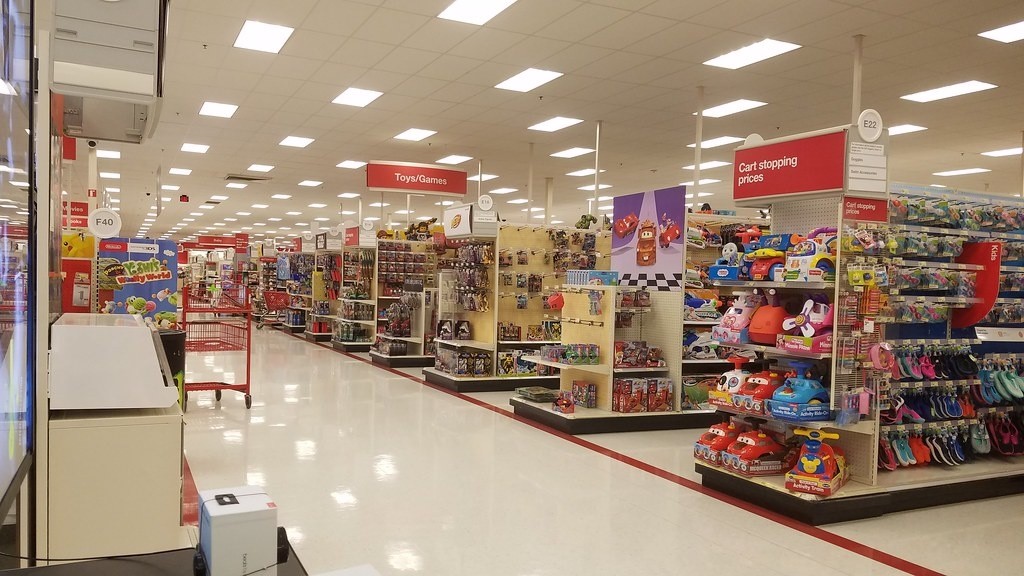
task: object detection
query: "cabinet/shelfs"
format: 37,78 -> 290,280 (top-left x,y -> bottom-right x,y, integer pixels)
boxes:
497,338 -> 561,391
330,298 -> 376,352
422,339 -> 494,392
185,249 -> 342,343
681,213 -> 771,414
696,280 -> 879,526
369,334 -> 422,368
521,284 -> 670,412
377,296 -> 400,321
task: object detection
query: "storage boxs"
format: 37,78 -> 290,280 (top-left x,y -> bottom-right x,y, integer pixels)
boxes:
435,347 -> 492,378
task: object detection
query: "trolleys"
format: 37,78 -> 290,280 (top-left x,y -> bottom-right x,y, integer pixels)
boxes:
256,288 -> 289,329
177,281 -> 254,410
